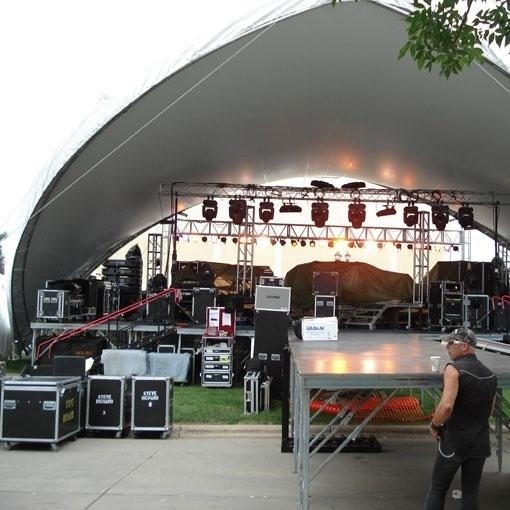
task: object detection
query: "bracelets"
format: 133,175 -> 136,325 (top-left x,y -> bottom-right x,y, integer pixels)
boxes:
430,421 -> 444,430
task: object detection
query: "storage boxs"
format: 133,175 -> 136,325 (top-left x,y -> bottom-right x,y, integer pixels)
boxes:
131,376 -> 173,439
84,375 -> 131,438
301,316 -> 339,341
0,375 -> 81,451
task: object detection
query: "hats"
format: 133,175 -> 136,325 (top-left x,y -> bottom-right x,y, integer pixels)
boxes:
439,327 -> 477,347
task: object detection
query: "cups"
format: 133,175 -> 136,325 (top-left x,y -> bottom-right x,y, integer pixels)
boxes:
430,356 -> 442,372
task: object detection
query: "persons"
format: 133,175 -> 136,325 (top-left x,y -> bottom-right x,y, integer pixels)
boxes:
425,327 -> 497,510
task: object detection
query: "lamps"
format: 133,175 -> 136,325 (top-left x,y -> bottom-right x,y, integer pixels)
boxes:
280,206 -> 302,214
404,207 -> 418,227
259,202 -> 274,223
432,206 -> 449,230
300,241 -> 306,247
348,204 -> 366,228
312,203 -> 329,227
221,237 -> 226,243
310,241 -> 315,248
458,207 -> 473,229
408,245 -> 412,249
348,241 -> 354,248
202,196 -> 217,221
396,244 -> 401,249
358,242 -> 364,248
378,243 -> 382,248
377,207 -> 396,218
271,239 -> 276,246
202,237 -> 207,243
280,240 -> 286,246
229,200 -> 246,224
328,242 -> 334,247
453,246 -> 458,251
291,240 -> 297,247
233,238 -> 237,244
